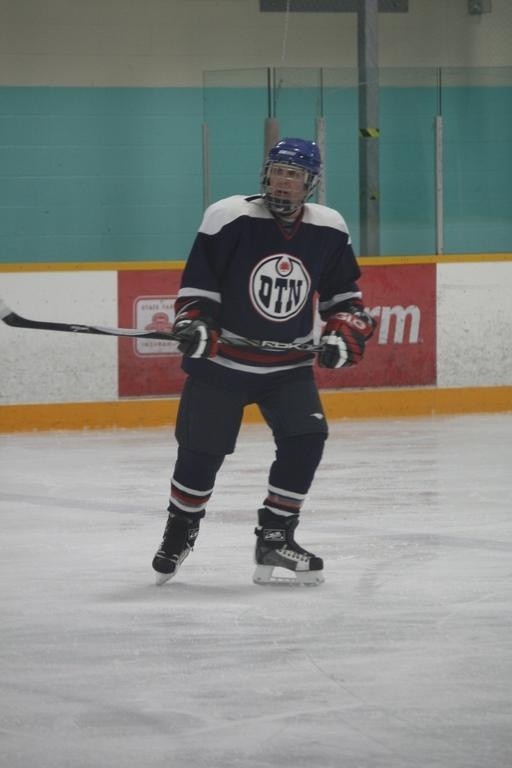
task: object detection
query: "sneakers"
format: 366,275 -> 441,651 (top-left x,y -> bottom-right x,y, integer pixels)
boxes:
253,505 -> 324,573
151,500 -> 207,575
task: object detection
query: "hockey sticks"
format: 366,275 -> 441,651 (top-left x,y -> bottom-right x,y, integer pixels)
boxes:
0,298 -> 326,352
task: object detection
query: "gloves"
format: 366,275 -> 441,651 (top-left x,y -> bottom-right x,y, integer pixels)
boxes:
322,314 -> 374,370
175,310 -> 224,360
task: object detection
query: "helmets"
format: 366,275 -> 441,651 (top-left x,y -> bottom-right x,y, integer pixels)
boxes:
259,135 -> 325,216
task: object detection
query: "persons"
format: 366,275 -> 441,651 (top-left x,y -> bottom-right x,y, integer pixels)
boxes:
149,137 -> 374,572
